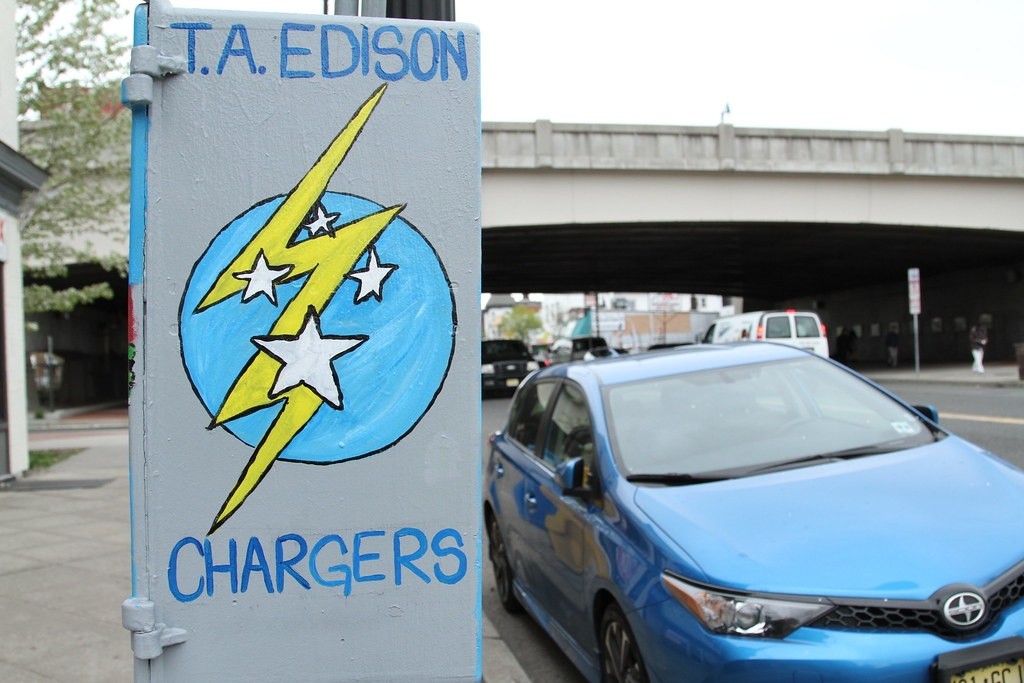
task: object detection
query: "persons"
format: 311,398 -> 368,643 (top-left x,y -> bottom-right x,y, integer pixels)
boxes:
969,314 -> 991,373
835,328 -> 849,365
886,326 -> 898,369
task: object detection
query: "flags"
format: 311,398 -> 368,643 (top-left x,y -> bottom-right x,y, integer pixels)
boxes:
571,311 -> 592,336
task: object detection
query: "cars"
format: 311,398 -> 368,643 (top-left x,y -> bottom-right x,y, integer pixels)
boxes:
483,342 -> 1023,683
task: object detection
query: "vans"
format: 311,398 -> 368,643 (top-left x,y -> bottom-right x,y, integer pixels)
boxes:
547,337 -> 611,365
700,307 -> 830,358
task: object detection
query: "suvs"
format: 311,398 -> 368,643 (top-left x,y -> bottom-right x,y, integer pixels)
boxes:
481,337 -> 541,397
